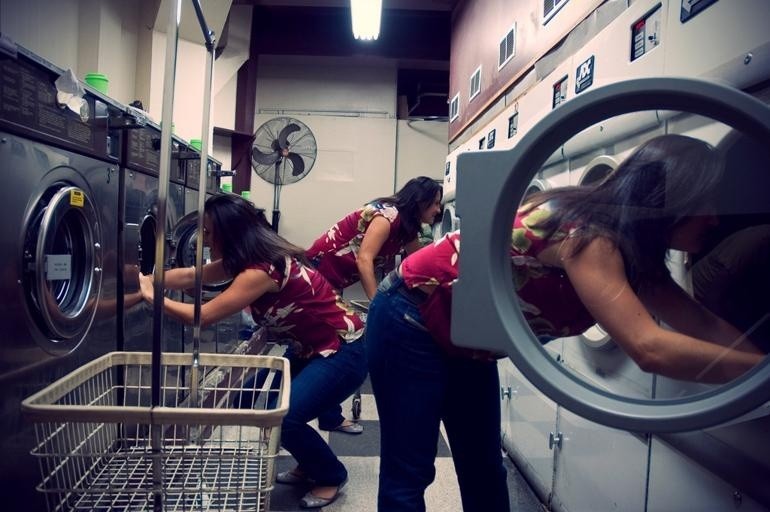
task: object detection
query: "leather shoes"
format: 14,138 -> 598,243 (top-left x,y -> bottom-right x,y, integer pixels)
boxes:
299,472 -> 350,508
275,469 -> 296,485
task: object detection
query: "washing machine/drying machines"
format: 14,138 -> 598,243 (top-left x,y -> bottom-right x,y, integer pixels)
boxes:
1,31 -> 243,512
442,0 -> 769,512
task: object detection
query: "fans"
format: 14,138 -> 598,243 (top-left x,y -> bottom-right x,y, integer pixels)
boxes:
248,116 -> 318,234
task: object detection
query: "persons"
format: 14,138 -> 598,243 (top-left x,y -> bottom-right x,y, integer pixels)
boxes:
139,190 -> 370,509
363,133 -> 767,511
304,175 -> 443,435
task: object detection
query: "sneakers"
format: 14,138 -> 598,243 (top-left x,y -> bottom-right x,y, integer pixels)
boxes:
333,420 -> 364,434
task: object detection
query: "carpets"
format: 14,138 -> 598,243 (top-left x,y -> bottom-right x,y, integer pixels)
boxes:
329,418 -> 452,458
